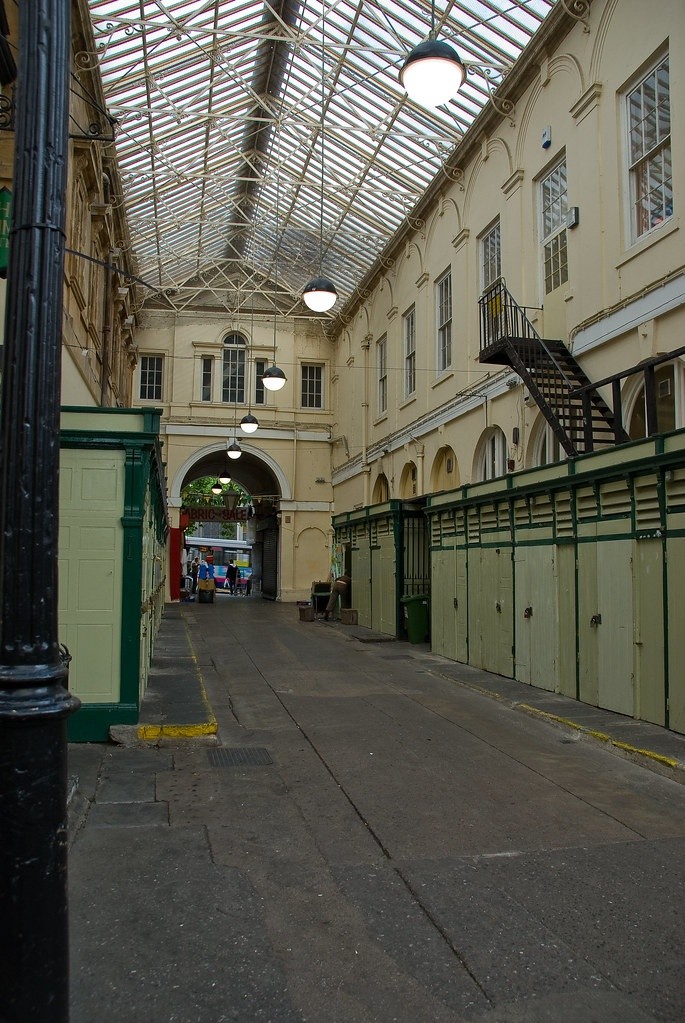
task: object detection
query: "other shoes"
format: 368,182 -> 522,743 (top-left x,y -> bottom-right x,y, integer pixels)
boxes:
324,611 -> 329,621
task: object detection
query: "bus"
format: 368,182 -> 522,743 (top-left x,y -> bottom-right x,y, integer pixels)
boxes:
185,535 -> 252,588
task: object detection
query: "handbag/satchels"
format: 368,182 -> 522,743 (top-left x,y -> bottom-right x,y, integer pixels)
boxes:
224,579 -> 230,588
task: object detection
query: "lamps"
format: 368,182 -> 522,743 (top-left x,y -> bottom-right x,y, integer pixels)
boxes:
221,484 -> 242,512
219,465 -> 232,484
566,206 -> 580,228
226,290 -> 242,460
397,0 -> 465,106
212,479 -> 223,495
301,0 -> 339,313
239,225 -> 260,433
261,120 -> 287,392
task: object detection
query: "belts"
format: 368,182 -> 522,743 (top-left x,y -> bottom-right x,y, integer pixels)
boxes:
337,580 -> 347,585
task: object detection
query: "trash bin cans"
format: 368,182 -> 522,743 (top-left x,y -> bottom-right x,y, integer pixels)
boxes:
400,594 -> 431,644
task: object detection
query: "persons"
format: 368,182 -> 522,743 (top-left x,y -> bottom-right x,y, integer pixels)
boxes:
322,571 -> 351,621
198,560 -> 208,578
191,556 -> 200,594
245,574 -> 253,596
226,559 -> 237,594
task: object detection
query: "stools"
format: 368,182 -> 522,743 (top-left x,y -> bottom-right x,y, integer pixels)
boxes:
299,606 -> 314,622
340,608 -> 357,625
